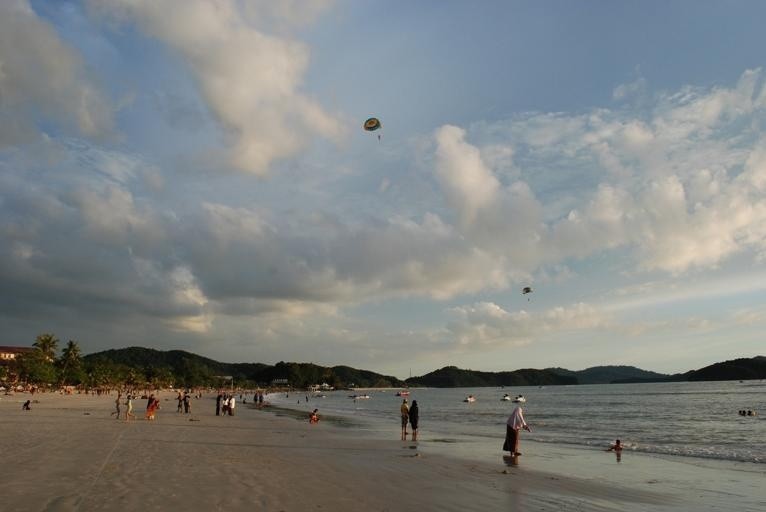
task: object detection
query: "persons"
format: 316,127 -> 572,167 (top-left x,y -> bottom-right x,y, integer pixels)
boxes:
122,394 -> 136,421
310,409 -> 320,424
742,410 -> 747,416
145,393 -> 161,421
198,389 -> 202,398
503,405 -> 532,456
747,410 -> 752,415
174,391 -> 192,415
601,438 -> 623,452
216,392 -> 235,417
253,392 -> 263,410
30,385 -> 36,395
23,399 -> 31,411
408,400 -> 420,439
399,397 -> 410,441
738,409 -> 742,415
111,393 -> 122,420
240,393 -> 243,403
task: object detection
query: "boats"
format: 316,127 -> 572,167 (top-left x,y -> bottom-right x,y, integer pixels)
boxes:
500,394 -> 525,402
350,395 -> 370,401
397,390 -> 409,396
463,394 -> 477,403
